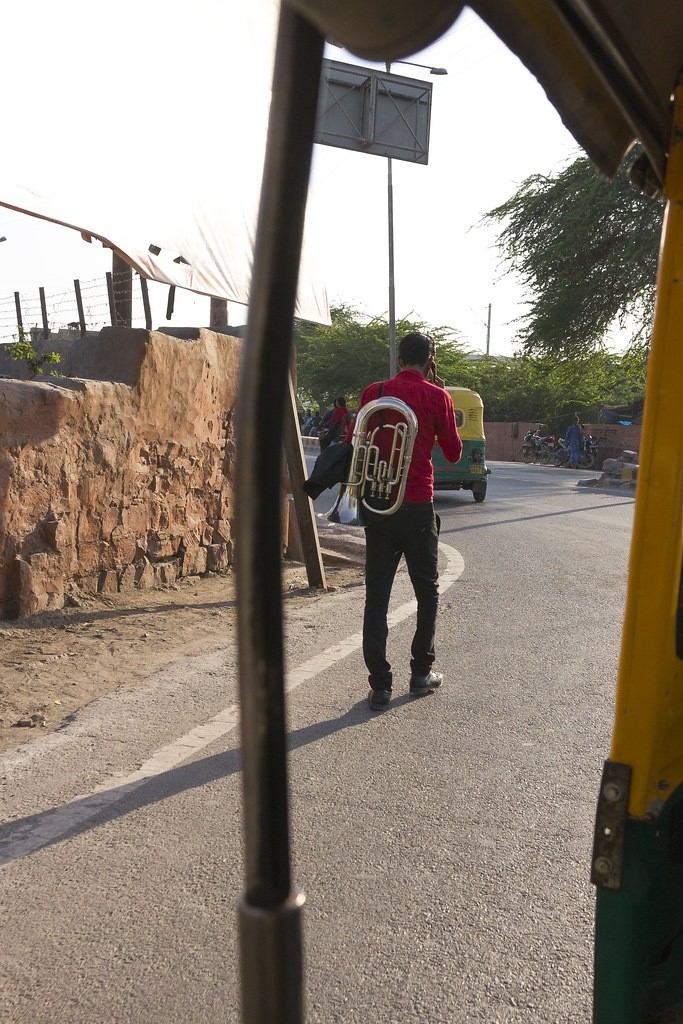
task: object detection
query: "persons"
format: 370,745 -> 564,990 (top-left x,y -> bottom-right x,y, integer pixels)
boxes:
303,397 -> 350,454
345,332 -> 462,711
565,416 -> 582,469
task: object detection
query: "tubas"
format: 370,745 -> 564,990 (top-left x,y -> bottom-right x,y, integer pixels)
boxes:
315,395 -> 420,529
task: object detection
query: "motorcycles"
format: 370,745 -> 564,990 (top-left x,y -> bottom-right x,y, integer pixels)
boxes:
431,382 -> 492,501
518,423 -> 599,470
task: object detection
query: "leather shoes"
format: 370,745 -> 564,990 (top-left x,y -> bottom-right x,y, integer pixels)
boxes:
410,670 -> 443,695
369,689 -> 392,710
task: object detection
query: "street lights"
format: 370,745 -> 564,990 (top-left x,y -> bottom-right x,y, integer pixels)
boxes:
382,57 -> 452,379
